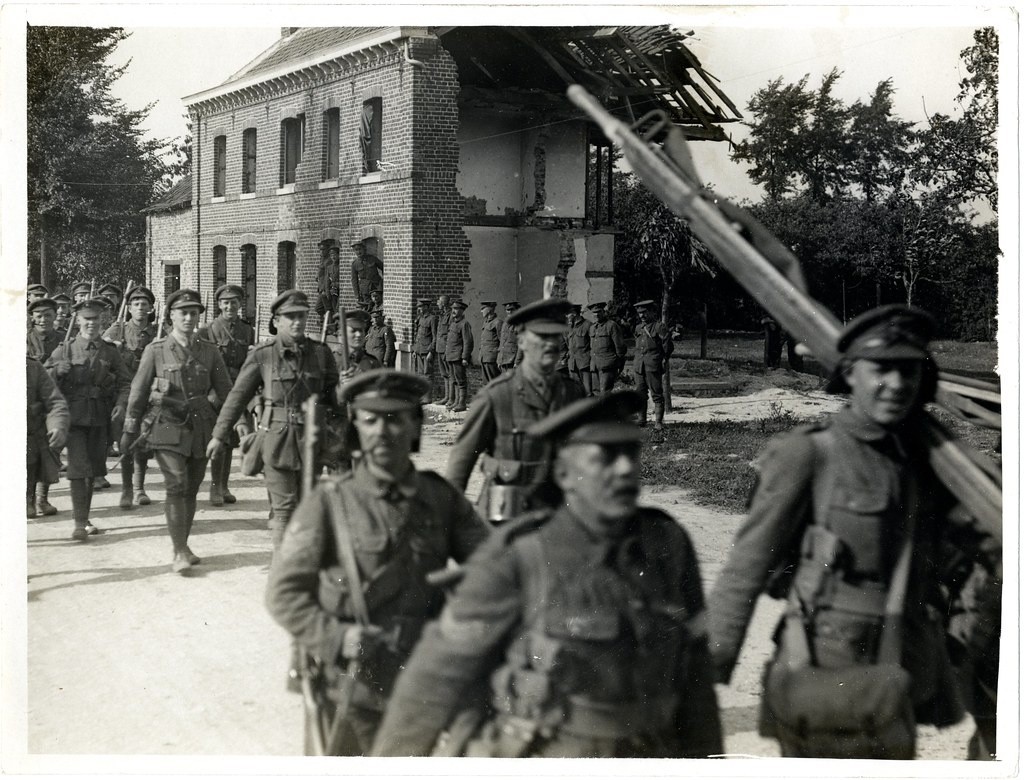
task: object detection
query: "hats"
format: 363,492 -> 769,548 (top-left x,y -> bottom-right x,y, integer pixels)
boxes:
166,289 -> 205,314
270,290 -> 310,315
527,389 -> 646,443
328,247 -> 339,254
215,285 -> 244,299
508,296 -> 572,334
567,300 -> 654,313
27,282 -> 123,318
318,243 -> 330,250
351,243 -> 363,249
837,306 -> 935,359
370,299 -> 520,317
339,369 -> 428,412
334,311 -> 371,329
127,286 -> 155,306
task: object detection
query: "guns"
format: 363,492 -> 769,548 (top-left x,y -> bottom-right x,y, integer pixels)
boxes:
156,305 -> 168,340
118,279 -> 133,322
301,394 -> 321,503
255,305 -> 260,345
90,278 -> 98,298
321,310 -> 331,343
64,311 -> 77,342
204,291 -> 208,324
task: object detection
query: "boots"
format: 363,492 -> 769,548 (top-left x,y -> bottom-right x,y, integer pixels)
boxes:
27,447 -> 236,572
436,378 -> 467,411
654,403 -> 665,429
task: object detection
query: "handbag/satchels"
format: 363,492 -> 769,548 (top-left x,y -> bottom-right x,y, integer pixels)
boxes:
238,432 -> 267,476
772,664 -> 915,760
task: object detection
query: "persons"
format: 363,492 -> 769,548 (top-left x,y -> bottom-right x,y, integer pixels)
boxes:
26,299 -> 65,518
27,283 -> 123,337
120,289 -> 250,572
478,301 -> 503,384
632,300 -> 671,430
350,242 -> 383,311
708,304 -> 1003,760
412,294 -> 474,411
565,304 -> 593,389
587,301 -> 626,395
205,289 -> 340,549
444,300 -> 588,525
43,300 -> 132,541
265,368 -> 489,756
102,286 -> 168,508
27,353 -> 70,484
315,243 -> 339,336
496,301 -> 523,373
376,388 -> 724,758
332,310 -> 383,477
197,284 -> 256,506
365,310 -> 394,367
371,290 -> 383,311
